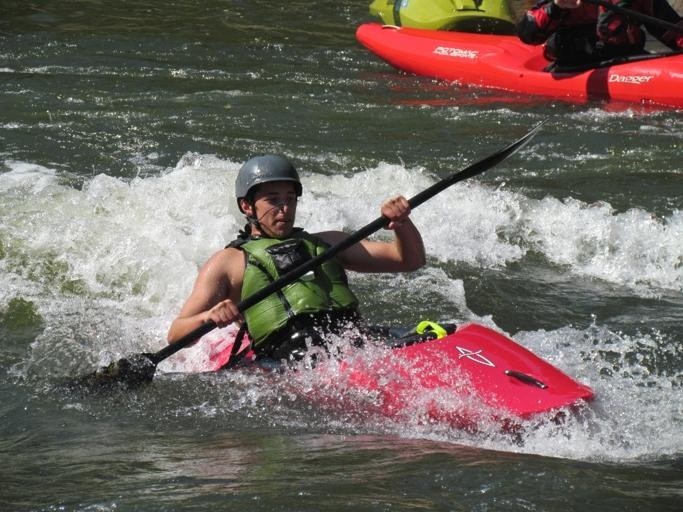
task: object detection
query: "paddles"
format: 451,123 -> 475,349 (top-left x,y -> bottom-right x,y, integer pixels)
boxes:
75,117 -> 550,397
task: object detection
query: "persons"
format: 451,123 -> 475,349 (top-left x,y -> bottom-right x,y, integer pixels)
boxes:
165,153 -> 428,359
516,0 -> 683,72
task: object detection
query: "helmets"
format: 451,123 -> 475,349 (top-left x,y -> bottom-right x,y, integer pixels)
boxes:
234,153 -> 304,203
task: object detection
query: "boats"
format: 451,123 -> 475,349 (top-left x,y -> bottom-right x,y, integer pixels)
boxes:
370,1 -> 517,32
357,24 -> 683,110
209,320 -> 593,430
